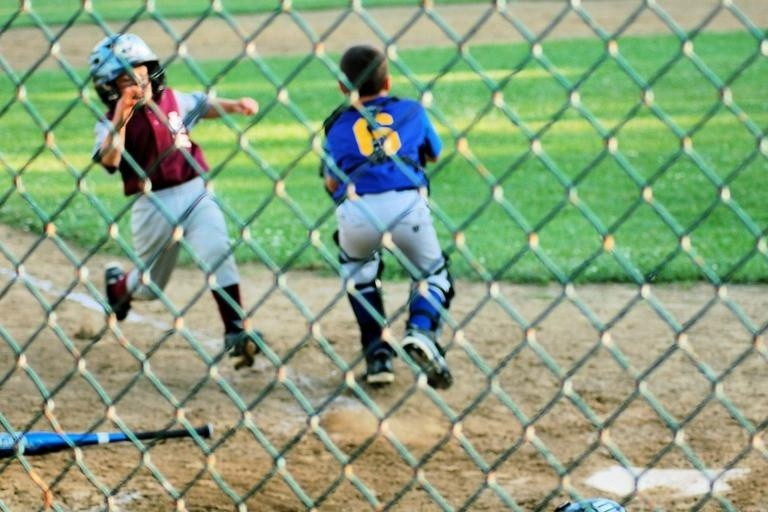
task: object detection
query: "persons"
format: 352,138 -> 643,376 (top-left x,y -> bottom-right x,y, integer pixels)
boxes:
319,44 -> 455,390
90,31 -> 266,370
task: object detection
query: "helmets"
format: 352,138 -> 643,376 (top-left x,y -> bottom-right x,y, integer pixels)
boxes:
87,34 -> 164,113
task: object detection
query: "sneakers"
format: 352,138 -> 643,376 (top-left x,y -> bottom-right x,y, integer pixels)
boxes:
366,348 -> 394,385
104,262 -> 131,321
402,321 -> 454,390
224,329 -> 264,371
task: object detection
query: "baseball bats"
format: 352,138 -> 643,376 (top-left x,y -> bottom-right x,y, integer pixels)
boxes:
1,424 -> 214,456
